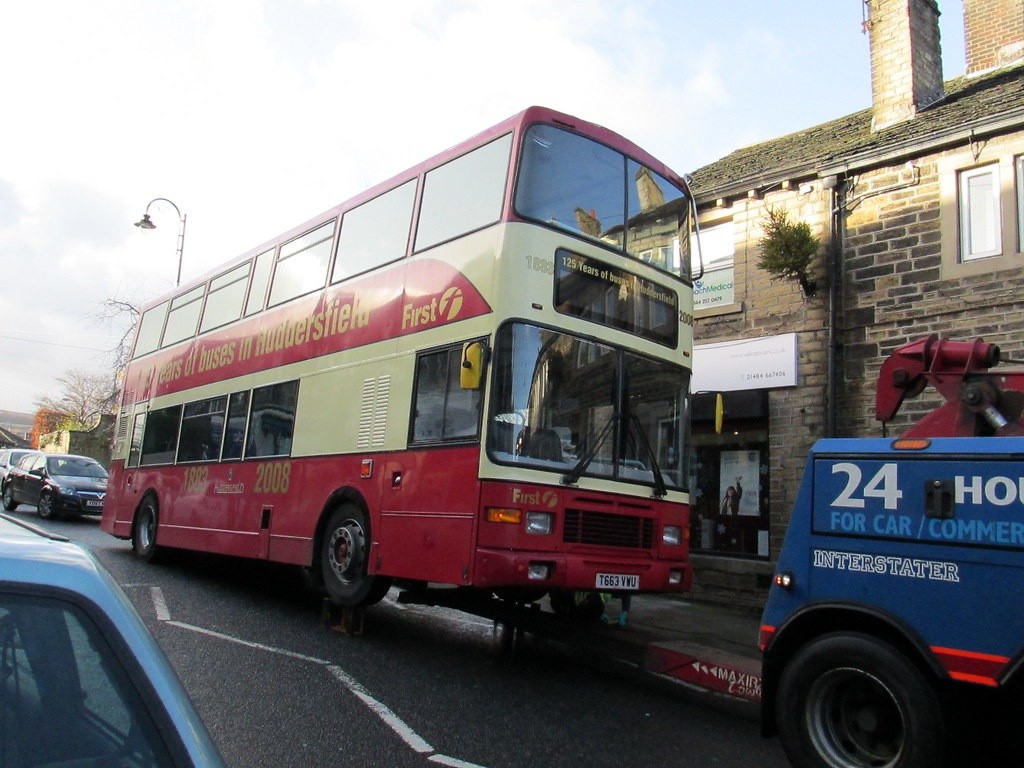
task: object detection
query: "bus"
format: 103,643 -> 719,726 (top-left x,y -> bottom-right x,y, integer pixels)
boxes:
100,106 -> 705,609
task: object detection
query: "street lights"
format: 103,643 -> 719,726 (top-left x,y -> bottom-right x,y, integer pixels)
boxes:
134,198 -> 186,287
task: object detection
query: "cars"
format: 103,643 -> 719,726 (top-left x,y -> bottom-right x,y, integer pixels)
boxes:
0,513 -> 226,768
0,449 -> 110,519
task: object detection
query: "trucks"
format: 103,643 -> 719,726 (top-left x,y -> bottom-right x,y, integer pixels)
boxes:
758,331 -> 1024,768
